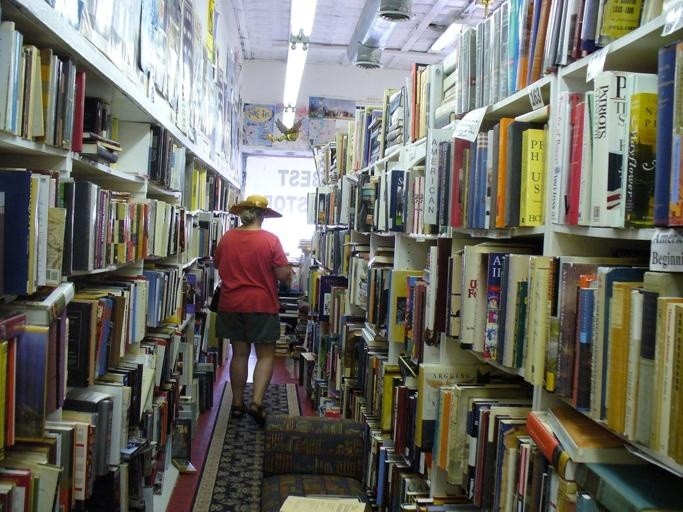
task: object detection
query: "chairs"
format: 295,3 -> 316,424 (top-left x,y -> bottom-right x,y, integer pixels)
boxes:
259,412 -> 371,511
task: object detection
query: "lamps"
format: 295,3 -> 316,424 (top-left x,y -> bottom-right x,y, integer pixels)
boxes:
271,0 -> 319,128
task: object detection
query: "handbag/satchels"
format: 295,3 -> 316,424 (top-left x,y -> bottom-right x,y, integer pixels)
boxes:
209,286 -> 222,313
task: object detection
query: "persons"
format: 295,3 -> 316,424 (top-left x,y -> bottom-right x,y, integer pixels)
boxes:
212,208 -> 291,425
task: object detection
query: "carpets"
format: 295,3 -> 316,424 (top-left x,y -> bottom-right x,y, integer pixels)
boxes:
164,380 -> 318,510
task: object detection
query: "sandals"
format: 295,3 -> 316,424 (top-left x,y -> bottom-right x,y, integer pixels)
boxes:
229,403 -> 248,419
246,401 -> 269,427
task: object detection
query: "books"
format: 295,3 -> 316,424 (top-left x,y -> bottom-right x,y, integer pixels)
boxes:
1,1 -> 241,511
282,0 -> 683,512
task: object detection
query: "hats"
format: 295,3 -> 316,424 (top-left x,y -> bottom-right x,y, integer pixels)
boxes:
228,194 -> 283,219
